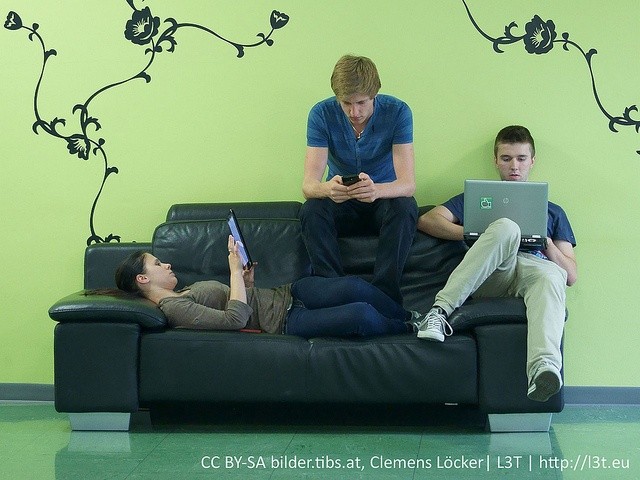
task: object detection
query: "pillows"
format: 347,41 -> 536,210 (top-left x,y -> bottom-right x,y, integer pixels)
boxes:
326,227 -> 467,310
446,298 -> 570,335
48,289 -> 169,333
151,216 -> 312,293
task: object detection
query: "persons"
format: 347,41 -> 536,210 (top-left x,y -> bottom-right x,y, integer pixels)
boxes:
113,234 -> 420,337
417,126 -> 577,400
299,54 -> 419,308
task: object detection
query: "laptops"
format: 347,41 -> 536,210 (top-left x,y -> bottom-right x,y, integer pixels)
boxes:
463,179 -> 548,252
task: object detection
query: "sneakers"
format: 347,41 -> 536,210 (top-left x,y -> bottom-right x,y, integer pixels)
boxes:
417,306 -> 454,343
527,362 -> 563,403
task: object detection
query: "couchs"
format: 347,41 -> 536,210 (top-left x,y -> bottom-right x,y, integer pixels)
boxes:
47,202 -> 569,433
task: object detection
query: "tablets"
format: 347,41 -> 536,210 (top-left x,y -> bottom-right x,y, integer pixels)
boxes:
225,208 -> 253,270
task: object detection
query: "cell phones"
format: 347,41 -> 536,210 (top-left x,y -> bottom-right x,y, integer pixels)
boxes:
342,175 -> 362,186
